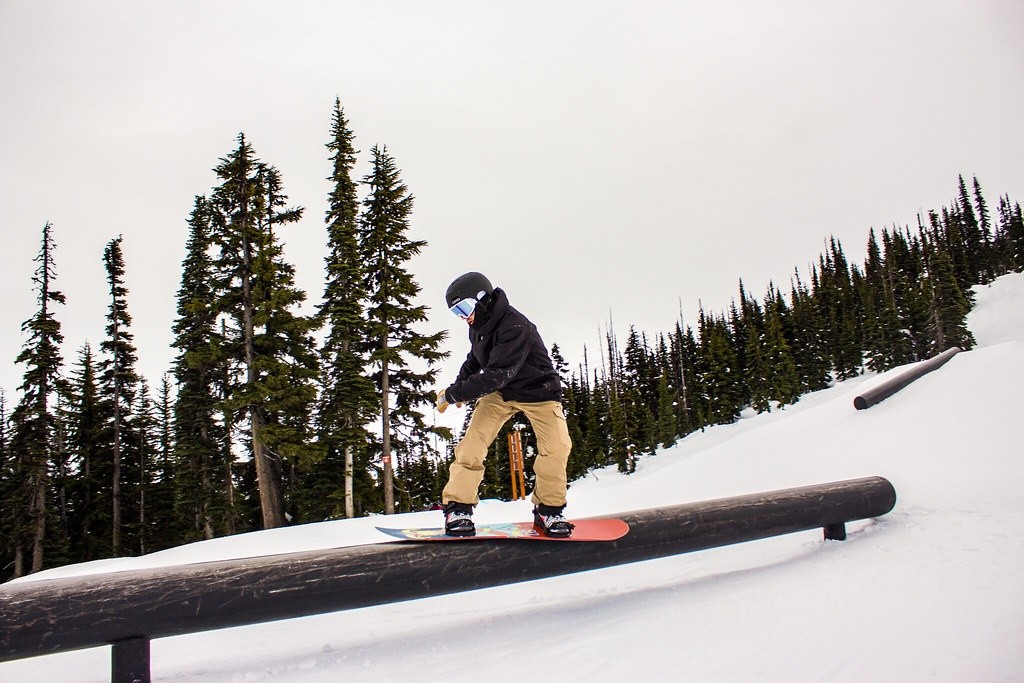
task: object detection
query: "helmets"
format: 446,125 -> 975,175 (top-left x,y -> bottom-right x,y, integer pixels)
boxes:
446,272 -> 493,310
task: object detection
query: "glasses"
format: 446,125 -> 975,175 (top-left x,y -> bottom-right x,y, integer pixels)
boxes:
449,291 -> 487,320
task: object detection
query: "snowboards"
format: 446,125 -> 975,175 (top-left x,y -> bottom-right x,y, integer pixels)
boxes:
375,517 -> 630,541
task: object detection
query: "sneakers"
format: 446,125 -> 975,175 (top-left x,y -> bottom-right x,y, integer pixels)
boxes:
443,503 -> 477,537
531,503 -> 575,538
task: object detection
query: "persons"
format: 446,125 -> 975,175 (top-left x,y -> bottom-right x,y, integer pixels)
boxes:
432,272 -> 576,537
